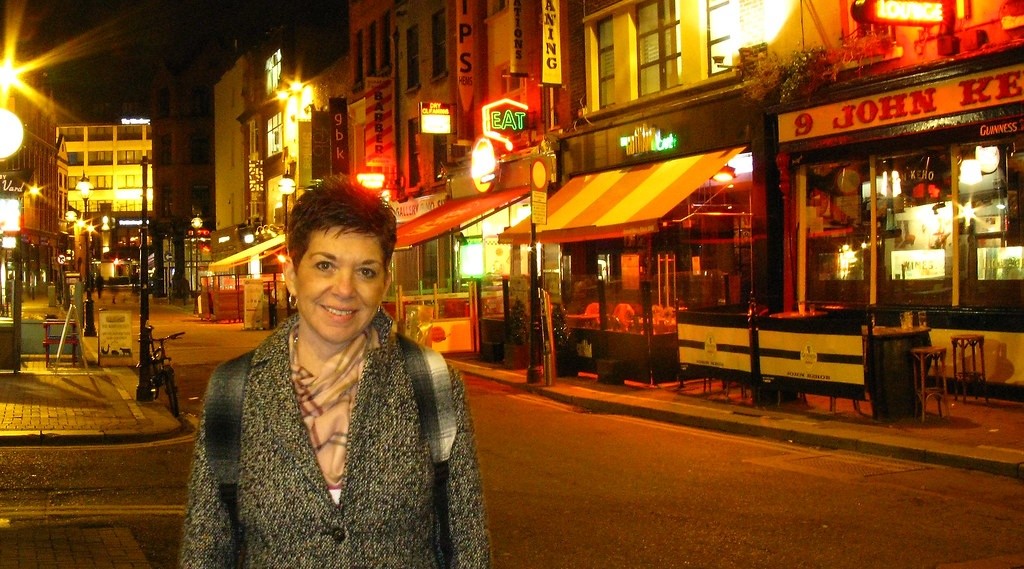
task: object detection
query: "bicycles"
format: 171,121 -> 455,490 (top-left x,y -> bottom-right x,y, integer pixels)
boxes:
135,323 -> 186,417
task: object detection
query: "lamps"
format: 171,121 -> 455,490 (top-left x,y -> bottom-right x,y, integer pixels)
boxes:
709,163 -> 737,182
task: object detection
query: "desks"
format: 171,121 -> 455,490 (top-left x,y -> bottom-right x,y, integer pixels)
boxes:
42,318 -> 78,368
863,327 -> 933,423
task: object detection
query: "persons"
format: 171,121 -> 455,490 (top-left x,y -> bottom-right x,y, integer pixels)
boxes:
178,172 -> 491,569
96,273 -> 105,298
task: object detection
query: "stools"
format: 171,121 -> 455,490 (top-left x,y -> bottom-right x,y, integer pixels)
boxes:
912,346 -> 951,423
951,334 -> 988,404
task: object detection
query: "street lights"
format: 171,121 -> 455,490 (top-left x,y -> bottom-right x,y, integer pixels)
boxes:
279,168 -> 296,319
76,170 -> 99,338
191,214 -> 203,315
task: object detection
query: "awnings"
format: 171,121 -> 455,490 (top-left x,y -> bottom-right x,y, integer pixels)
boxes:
497,146 -> 746,245
209,234 -> 288,271
394,184 -> 532,252
